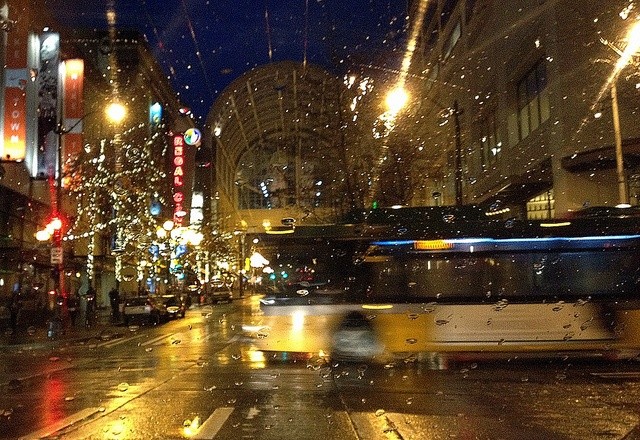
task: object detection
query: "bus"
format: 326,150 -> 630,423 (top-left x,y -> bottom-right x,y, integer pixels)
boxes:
256,205 -> 640,365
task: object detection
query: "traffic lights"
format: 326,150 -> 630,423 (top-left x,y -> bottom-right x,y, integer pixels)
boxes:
49,218 -> 61,234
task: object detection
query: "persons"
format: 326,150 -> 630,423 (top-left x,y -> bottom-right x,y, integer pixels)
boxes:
110,289 -> 121,323
84,290 -> 97,328
198,292 -> 205,307
185,293 -> 192,308
69,293 -> 80,327
55,296 -> 69,335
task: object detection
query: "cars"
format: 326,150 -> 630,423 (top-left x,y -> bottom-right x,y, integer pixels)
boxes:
209,288 -> 232,303
122,296 -> 169,326
155,294 -> 185,319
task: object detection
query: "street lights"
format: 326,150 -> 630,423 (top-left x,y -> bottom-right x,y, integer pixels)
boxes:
53,102 -> 127,323
384,85 -> 463,205
608,21 -> 640,206
238,219 -> 271,297
